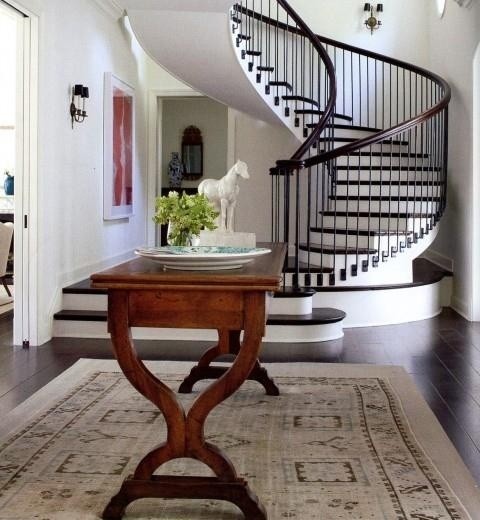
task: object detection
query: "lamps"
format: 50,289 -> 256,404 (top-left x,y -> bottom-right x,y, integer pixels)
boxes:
70,84 -> 89,123
363,3 -> 384,36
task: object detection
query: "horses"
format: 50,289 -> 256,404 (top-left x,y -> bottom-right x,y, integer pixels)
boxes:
196,157 -> 250,233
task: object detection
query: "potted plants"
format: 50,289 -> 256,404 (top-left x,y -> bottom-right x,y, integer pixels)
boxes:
151,190 -> 221,246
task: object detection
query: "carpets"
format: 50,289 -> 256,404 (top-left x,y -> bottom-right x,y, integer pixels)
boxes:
0,357 -> 478,520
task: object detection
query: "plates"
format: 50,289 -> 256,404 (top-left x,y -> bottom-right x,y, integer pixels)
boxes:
135,245 -> 273,271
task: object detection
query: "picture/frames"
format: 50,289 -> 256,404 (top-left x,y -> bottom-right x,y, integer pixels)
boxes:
102,71 -> 138,220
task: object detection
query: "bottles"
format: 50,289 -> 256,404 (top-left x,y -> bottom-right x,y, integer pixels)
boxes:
4,176 -> 13,195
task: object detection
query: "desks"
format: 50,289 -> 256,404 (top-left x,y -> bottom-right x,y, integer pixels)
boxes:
90,242 -> 287,519
